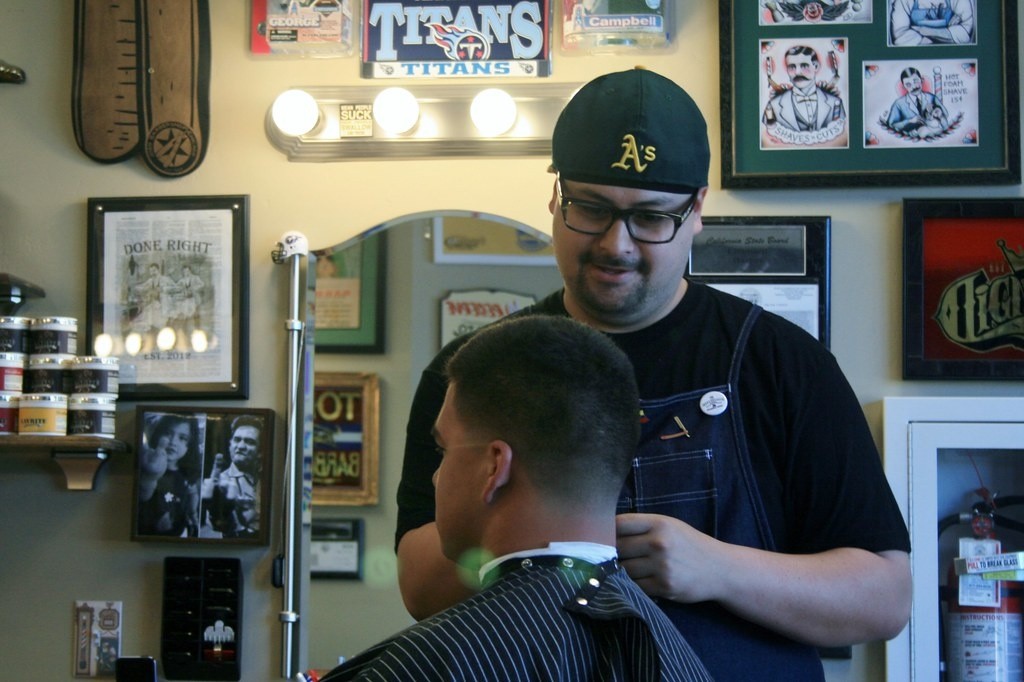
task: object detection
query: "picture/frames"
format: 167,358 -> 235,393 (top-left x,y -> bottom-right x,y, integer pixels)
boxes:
432,216 -> 557,267
718,0 -> 1019,188
127,404 -> 275,544
900,196 -> 1024,380
86,193 -> 252,399
314,231 -> 388,354
311,371 -> 381,506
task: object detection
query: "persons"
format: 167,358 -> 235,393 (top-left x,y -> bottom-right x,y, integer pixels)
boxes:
201,413 -> 263,502
315,313 -> 715,682
126,262 -> 204,343
389,65 -> 913,682
136,410 -> 200,538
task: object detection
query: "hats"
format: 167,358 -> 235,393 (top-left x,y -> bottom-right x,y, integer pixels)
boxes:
552,64 -> 711,195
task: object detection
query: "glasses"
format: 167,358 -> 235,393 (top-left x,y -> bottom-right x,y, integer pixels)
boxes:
555,171 -> 696,244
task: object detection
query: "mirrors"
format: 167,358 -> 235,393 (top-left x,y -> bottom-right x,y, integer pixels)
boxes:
296,208 -> 564,682
